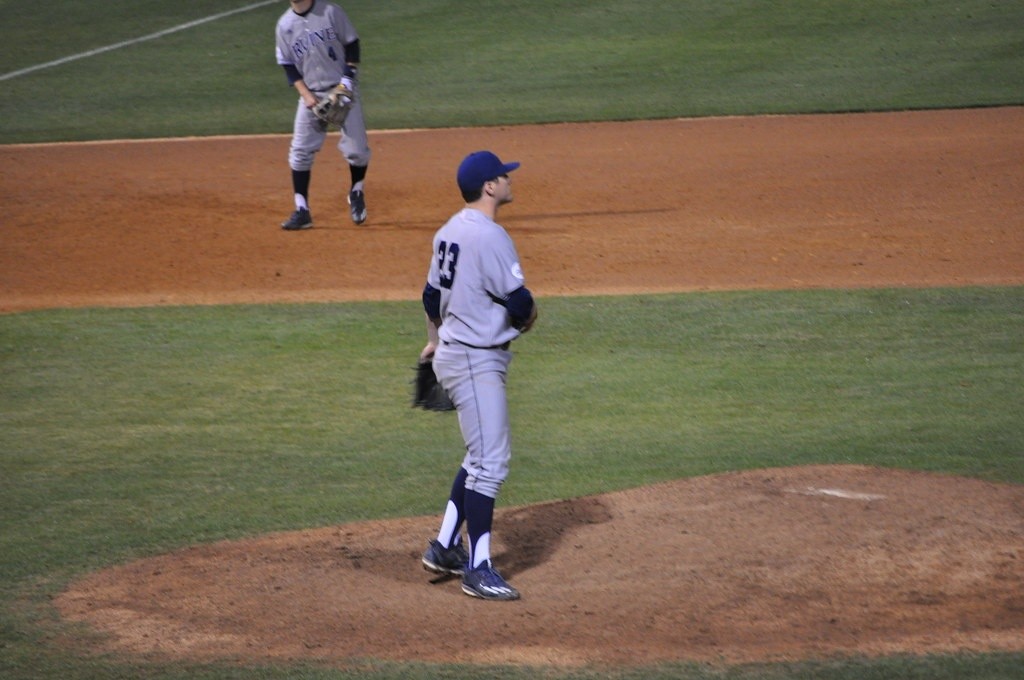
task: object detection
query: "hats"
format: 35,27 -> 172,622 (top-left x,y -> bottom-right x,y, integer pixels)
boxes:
458,150 -> 521,191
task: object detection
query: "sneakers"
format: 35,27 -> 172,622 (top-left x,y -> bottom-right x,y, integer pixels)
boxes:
422,536 -> 471,577
463,560 -> 521,600
281,206 -> 314,229
348,190 -> 368,222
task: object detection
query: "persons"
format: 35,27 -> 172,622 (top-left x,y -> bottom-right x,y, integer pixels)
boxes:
275,0 -> 371,231
419,150 -> 537,600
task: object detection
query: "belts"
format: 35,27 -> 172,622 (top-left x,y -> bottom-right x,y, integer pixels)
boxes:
443,340 -> 511,351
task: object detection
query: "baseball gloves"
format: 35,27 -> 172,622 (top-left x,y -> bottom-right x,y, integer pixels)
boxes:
413,356 -> 457,411
310,87 -> 355,126
